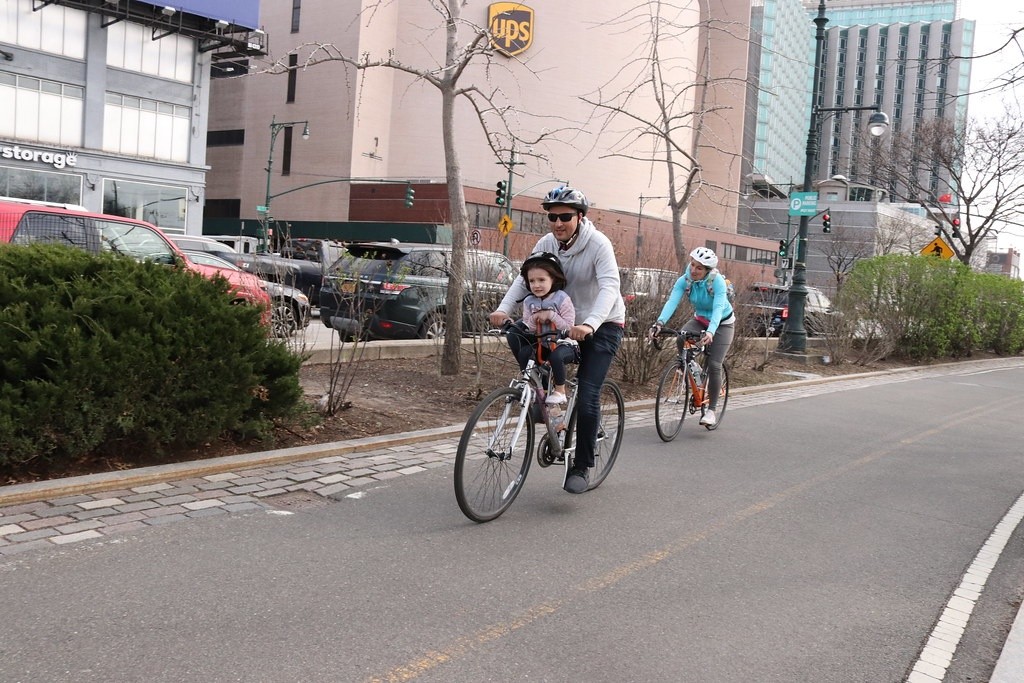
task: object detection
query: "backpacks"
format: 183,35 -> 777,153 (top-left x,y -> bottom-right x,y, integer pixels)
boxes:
686,268 -> 735,307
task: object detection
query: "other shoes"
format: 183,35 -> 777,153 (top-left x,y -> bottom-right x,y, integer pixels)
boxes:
545,391 -> 567,404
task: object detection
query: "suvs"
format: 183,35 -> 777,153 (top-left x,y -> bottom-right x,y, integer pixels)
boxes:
319,242 -> 522,343
0,199 -> 272,339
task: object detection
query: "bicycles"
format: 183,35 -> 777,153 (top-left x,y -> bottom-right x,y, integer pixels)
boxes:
653,323 -> 729,442
452,314 -> 626,524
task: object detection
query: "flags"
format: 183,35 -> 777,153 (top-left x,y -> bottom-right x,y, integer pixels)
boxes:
939,194 -> 951,202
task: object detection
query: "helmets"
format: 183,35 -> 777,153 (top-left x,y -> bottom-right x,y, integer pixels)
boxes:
690,246 -> 719,272
540,185 -> 588,217
520,250 -> 567,292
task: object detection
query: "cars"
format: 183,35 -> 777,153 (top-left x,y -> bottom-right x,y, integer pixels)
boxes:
617,267 -> 682,335
744,312 -> 785,337
0,195 -> 354,338
737,280 -> 849,339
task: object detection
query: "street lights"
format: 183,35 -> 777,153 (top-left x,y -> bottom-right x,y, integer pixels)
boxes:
261,114 -> 310,255
775,1 -> 890,356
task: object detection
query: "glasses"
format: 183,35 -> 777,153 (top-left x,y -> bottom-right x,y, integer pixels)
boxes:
547,212 -> 579,223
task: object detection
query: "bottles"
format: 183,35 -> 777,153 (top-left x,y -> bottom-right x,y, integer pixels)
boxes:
692,366 -> 701,386
689,359 -> 702,373
548,403 -> 564,426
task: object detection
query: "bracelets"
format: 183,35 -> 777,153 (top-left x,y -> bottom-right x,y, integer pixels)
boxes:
583,324 -> 594,333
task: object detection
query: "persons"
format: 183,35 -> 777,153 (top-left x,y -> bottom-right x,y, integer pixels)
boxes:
520,251 -> 580,404
648,247 -> 737,425
488,185 -> 626,494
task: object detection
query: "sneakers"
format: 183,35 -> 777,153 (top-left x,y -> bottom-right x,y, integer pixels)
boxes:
688,359 -> 703,387
699,409 -> 716,426
532,402 -> 546,424
564,465 -> 590,493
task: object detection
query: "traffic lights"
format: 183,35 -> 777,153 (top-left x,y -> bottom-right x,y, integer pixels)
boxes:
822,214 -> 831,233
495,180 -> 507,205
406,187 -> 415,208
934,225 -> 943,236
779,239 -> 787,257
952,218 -> 960,238
